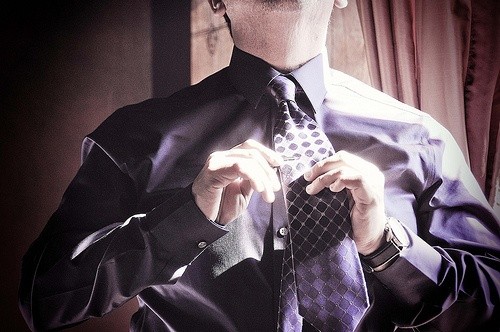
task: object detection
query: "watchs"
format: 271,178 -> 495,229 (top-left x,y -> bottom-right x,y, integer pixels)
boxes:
367,216 -> 410,269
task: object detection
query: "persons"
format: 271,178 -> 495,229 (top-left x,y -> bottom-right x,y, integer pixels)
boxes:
1,0 -> 500,332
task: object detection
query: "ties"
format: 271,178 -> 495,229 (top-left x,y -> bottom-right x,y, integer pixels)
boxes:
261,73 -> 372,332
